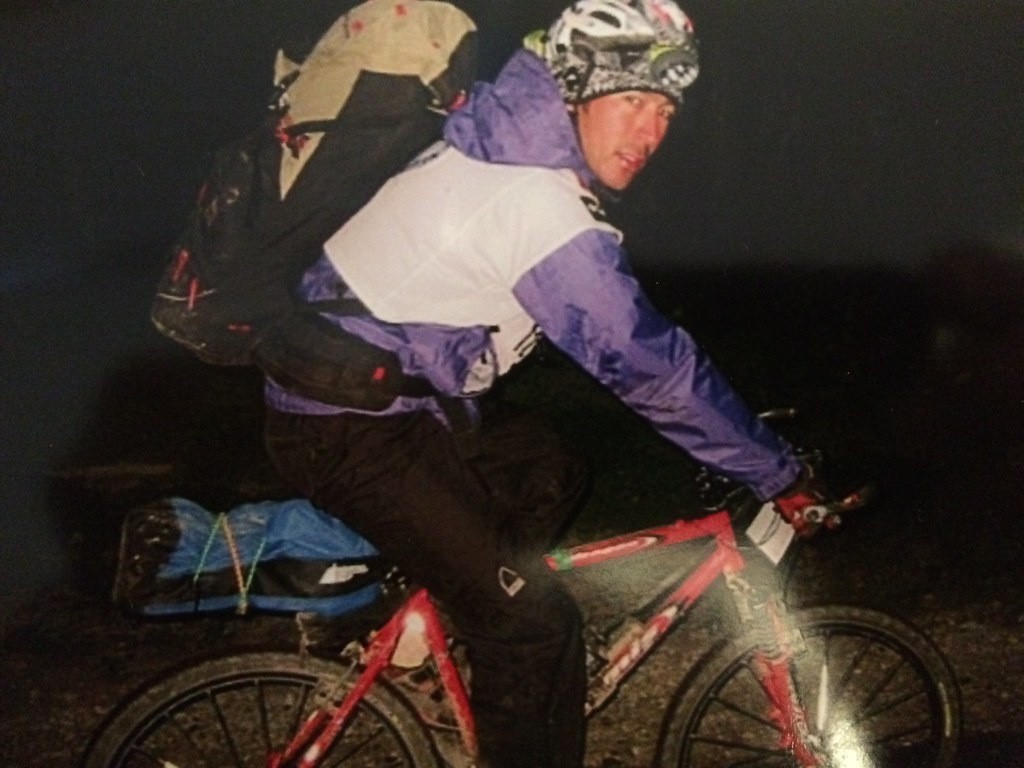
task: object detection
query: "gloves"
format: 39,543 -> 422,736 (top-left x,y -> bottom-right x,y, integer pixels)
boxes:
776,472 -> 845,541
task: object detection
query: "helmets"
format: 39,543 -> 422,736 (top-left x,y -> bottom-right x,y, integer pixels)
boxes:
544,0 -> 701,114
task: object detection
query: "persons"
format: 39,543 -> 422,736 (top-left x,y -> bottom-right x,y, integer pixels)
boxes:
228,1 -> 891,768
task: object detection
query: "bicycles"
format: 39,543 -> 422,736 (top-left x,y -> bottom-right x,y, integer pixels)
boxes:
78,394 -> 964,766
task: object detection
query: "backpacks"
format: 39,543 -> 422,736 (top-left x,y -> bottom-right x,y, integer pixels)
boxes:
151,0 -> 486,367
